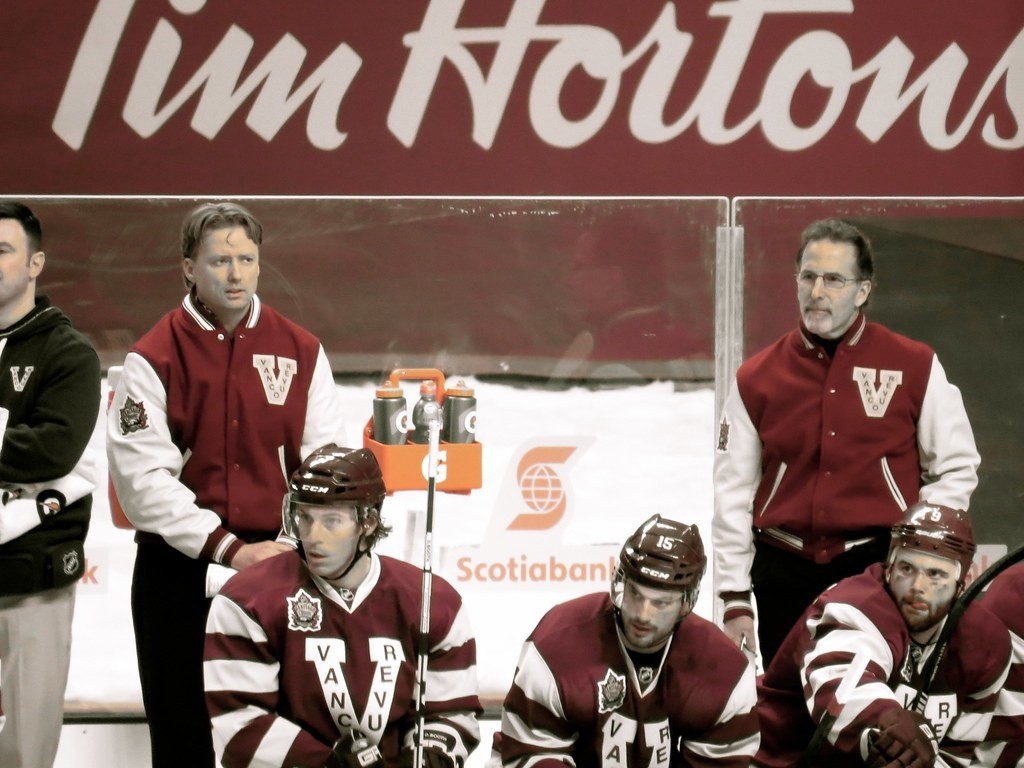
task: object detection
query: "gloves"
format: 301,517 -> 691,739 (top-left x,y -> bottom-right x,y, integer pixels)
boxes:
866,708 -> 937,767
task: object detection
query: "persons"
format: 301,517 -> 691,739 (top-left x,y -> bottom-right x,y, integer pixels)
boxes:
968,558 -> 1024,768
107,202 -> 345,768
0,202 -> 102,768
758,500 -> 1014,768
489,515 -> 761,768
202,443 -> 485,768
713,218 -> 981,677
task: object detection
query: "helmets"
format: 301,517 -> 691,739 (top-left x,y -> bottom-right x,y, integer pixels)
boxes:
609,512 -> 707,589
885,500 -> 976,583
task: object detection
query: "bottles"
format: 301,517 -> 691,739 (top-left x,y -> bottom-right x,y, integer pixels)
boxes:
441,381 -> 477,444
412,381 -> 442,444
373,381 -> 407,444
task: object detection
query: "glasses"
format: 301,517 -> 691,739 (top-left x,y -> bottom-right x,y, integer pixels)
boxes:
797,271 -> 863,290
282,443 -> 386,503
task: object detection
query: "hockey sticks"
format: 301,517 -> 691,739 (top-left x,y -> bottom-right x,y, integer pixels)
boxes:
412,420 -> 442,768
911,541 -> 1023,716
